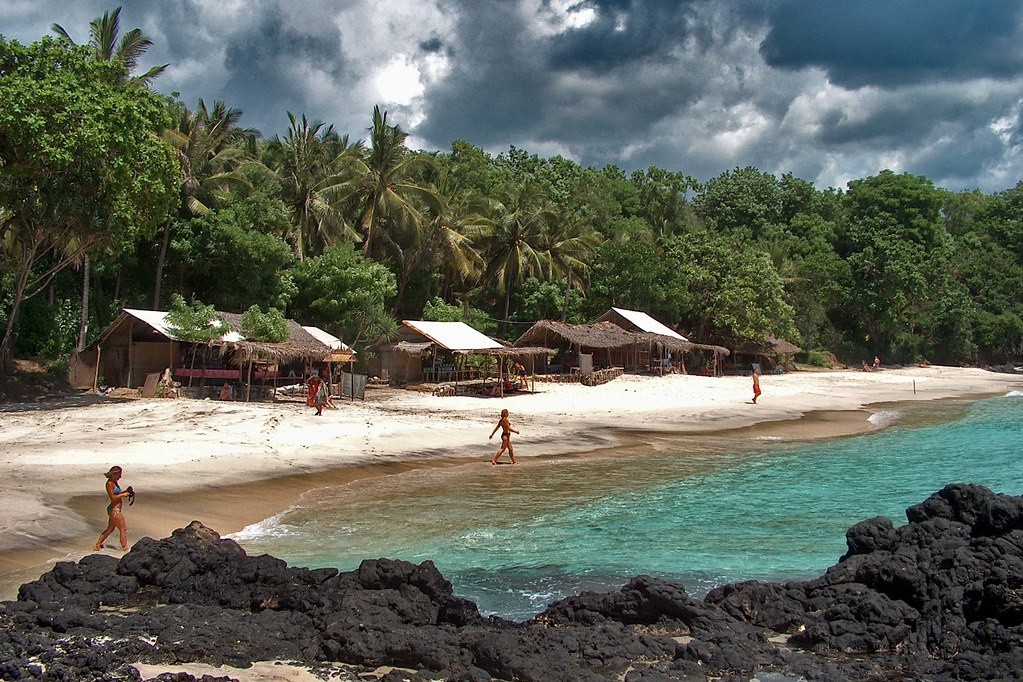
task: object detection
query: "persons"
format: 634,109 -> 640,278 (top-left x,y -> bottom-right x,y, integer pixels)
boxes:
515,363 -> 528,390
503,378 -> 515,394
874,356 -> 880,372
307,372 -> 328,416
752,368 -> 761,404
93,466 -> 134,553
863,362 -> 871,372
489,408 -> 520,466
490,385 -> 500,395
